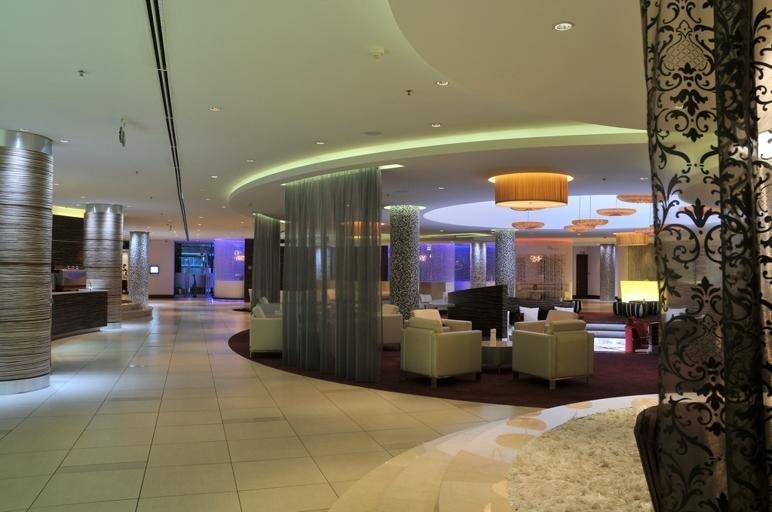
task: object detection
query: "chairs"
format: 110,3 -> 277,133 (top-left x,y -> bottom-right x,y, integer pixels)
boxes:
401,309 -> 484,383
583,296 -> 660,354
249,298 -> 403,354
512,310 -> 594,389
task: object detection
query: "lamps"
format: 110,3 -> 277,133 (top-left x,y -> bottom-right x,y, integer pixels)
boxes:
490,130 -> 771,247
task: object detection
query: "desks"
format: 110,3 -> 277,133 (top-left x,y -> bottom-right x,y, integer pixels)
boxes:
480,339 -> 512,373
